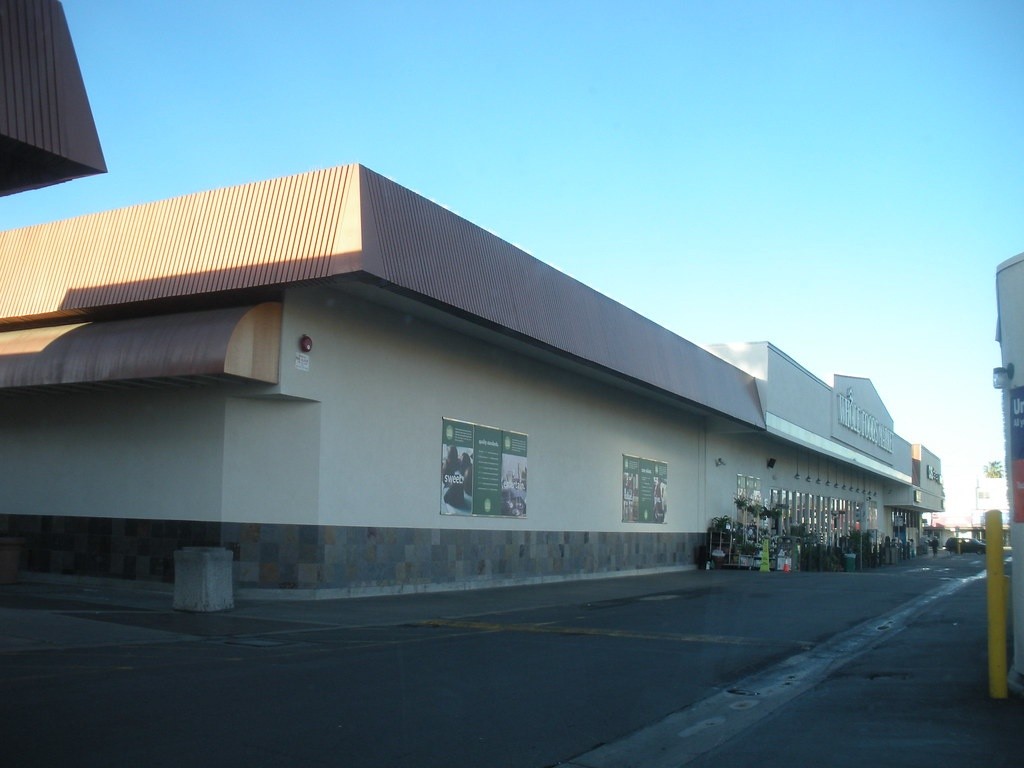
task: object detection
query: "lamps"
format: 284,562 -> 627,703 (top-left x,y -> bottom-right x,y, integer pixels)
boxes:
794,447 -> 878,496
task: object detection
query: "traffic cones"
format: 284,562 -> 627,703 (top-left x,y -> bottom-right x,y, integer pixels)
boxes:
782,557 -> 792,574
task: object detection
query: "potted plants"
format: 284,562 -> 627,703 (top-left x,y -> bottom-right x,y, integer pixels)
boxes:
712,495 -> 783,565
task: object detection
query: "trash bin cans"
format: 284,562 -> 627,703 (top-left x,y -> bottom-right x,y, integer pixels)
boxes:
844,553 -> 856,572
172,547 -> 235,613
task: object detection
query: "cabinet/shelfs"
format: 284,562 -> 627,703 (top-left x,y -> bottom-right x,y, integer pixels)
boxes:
709,531 -> 777,571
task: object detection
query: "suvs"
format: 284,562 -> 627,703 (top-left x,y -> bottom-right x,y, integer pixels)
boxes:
946,536 -> 985,556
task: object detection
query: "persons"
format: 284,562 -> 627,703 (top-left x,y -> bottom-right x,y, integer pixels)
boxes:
931,536 -> 939,558
910,539 -> 915,557
